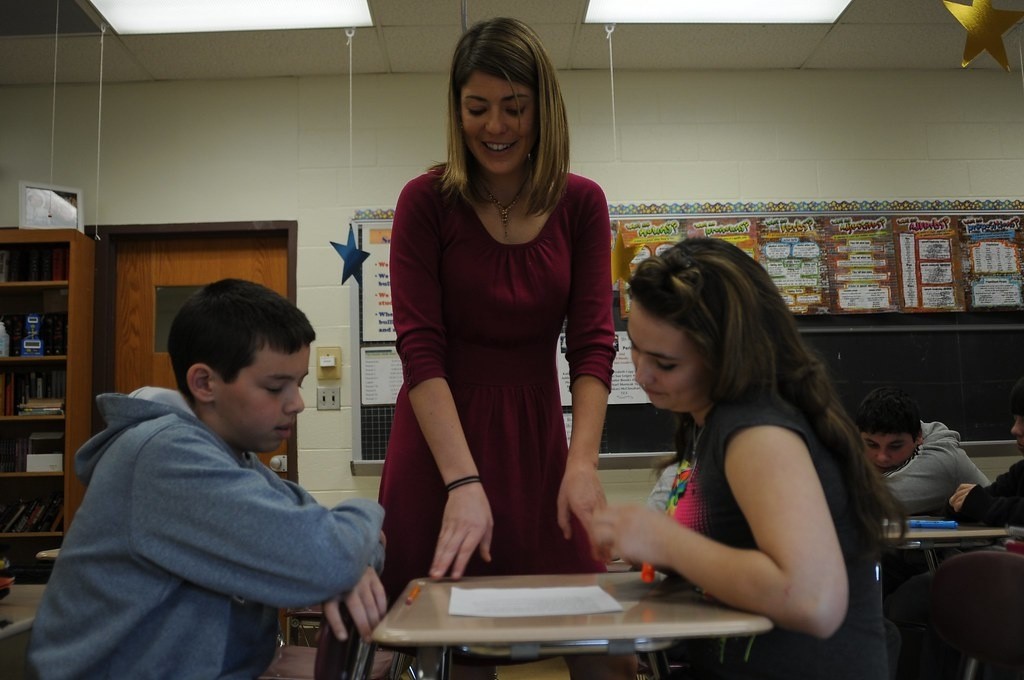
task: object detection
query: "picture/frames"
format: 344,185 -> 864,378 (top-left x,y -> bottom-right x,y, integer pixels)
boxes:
18,182 -> 84,233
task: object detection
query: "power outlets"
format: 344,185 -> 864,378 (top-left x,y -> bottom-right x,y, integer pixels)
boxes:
317,387 -> 341,410
316,348 -> 342,380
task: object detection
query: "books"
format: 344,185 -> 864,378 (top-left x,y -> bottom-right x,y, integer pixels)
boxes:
0,243 -> 70,584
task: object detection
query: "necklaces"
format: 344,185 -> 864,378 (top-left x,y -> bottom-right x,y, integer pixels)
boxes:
471,180 -> 533,239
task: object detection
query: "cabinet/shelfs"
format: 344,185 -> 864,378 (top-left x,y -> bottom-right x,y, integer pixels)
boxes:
0,229 -> 97,572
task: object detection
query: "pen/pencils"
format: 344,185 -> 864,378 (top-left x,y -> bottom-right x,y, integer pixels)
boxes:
405,586 -> 421,605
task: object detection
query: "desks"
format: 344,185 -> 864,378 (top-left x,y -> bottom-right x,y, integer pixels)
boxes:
370,571 -> 772,680
878,515 -> 1013,574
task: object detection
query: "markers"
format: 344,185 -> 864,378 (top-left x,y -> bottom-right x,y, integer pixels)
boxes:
908,519 -> 958,529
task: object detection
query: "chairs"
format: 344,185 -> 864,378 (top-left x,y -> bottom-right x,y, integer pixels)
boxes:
932,551 -> 1024,680
313,603 -> 375,680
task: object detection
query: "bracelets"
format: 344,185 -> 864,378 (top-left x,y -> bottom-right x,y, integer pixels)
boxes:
446,472 -> 482,492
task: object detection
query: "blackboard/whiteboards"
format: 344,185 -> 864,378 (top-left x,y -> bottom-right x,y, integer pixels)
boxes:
349,198 -> 1024,476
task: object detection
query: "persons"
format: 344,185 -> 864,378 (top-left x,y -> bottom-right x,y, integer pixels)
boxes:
594,235 -> 1024,679
377,17 -> 639,680
28,277 -> 387,680
